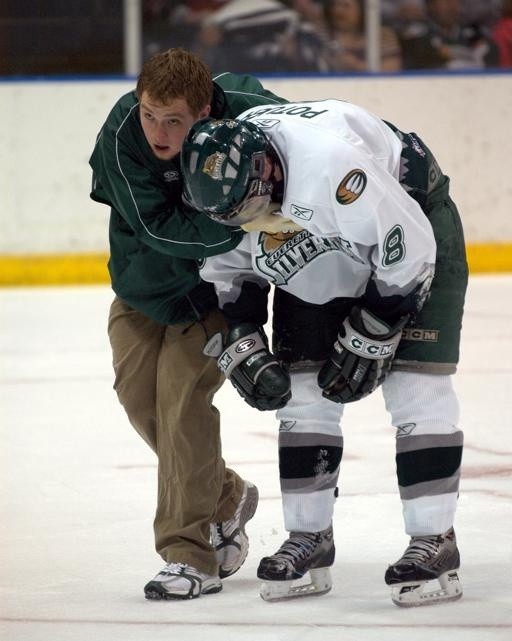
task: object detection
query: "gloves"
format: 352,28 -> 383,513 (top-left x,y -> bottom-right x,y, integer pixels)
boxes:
239,203 -> 304,234
201,322 -> 293,412
317,305 -> 410,407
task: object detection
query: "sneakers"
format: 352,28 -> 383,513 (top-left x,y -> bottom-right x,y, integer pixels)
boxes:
143,562 -> 224,603
208,481 -> 259,580
383,526 -> 462,587
257,519 -> 336,584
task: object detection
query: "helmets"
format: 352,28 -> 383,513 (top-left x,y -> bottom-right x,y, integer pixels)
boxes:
181,116 -> 269,215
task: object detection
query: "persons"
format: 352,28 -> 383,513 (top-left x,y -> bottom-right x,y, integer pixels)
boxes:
86,47 -> 288,600
109,1 -> 512,76
180,98 -> 469,585
